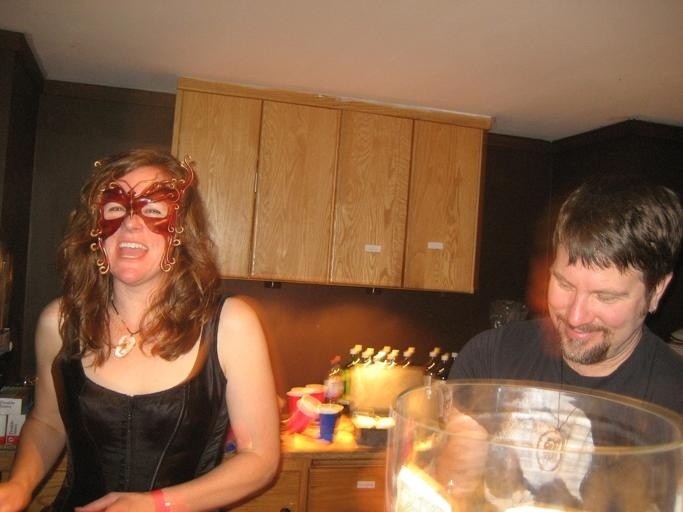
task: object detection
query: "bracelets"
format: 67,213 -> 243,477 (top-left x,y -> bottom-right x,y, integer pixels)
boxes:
150,488 -> 168,512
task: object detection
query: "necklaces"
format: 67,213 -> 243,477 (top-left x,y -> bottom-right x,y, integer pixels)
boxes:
111,299 -> 151,357
534,350 -> 590,472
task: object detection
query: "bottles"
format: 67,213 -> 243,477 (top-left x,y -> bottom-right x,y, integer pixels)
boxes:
345,343 -> 418,367
326,356 -> 346,403
425,347 -> 459,384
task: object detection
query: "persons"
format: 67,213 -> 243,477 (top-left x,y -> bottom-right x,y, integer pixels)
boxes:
423,172 -> 683,511
0,150 -> 282,511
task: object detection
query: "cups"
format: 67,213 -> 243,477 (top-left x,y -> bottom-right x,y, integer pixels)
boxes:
286,384 -> 343,443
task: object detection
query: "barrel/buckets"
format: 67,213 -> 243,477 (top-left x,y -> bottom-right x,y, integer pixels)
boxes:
390,379 -> 683,512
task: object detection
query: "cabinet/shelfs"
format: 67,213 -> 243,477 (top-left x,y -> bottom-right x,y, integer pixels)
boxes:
176,88 -> 342,283
330,111 -> 484,294
